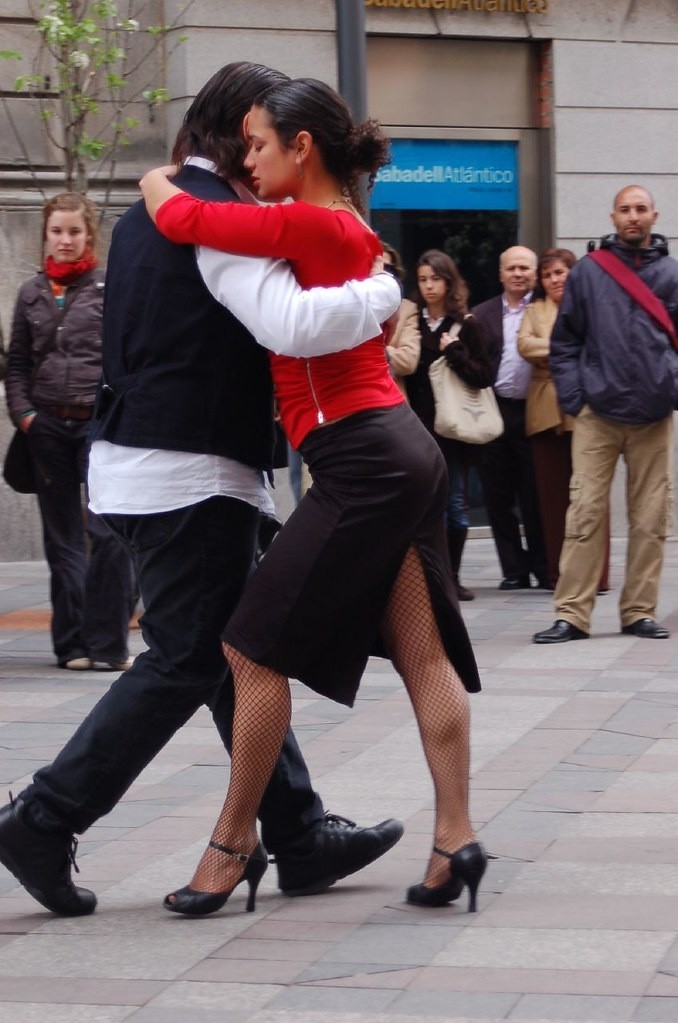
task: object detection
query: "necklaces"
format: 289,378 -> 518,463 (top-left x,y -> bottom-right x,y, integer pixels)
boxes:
325,199 -> 351,208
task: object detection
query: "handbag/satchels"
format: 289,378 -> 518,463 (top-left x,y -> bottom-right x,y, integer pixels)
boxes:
2,427 -> 38,495
428,313 -> 505,445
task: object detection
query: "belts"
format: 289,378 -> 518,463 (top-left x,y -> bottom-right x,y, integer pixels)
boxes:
41,405 -> 92,421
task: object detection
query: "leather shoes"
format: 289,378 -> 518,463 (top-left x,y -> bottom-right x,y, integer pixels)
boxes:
0,790 -> 98,917
534,620 -> 590,643
621,618 -> 671,638
268,809 -> 405,896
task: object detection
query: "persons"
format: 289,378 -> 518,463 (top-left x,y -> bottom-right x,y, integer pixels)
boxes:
7,191 -> 139,671
531,185 -> 678,643
0,64 -> 403,916
140,79 -> 487,917
375,239 -> 610,601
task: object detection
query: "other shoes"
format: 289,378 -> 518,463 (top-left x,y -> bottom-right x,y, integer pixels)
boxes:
92,655 -> 136,670
457,583 -> 475,601
500,576 -> 530,589
66,657 -> 92,670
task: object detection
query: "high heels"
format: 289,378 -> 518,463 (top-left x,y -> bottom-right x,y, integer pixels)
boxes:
406,840 -> 488,912
162,841 -> 269,917
